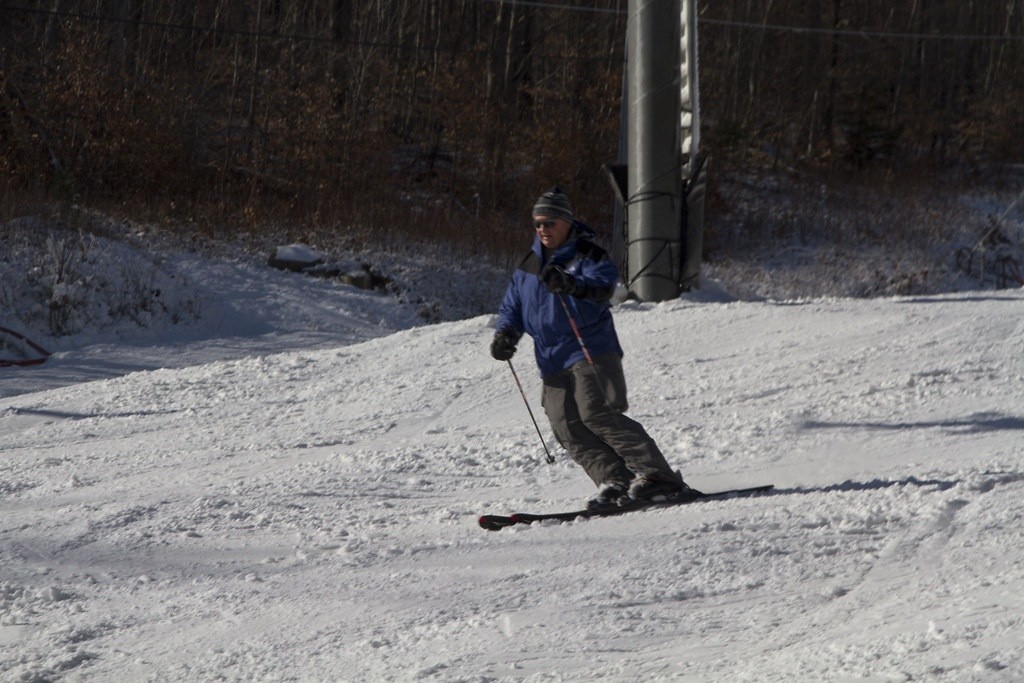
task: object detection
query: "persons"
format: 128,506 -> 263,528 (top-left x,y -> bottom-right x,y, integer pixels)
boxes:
491,192 -> 686,510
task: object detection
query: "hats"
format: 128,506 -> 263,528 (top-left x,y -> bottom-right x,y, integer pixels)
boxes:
533,193 -> 572,222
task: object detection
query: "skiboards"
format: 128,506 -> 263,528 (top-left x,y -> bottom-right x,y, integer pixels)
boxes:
478,485 -> 776,531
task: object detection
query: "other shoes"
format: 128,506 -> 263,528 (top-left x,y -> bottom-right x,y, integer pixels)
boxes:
586,482 -> 629,508
628,474 -> 684,501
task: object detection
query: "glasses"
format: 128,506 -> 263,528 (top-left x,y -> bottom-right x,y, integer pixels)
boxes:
533,220 -> 567,229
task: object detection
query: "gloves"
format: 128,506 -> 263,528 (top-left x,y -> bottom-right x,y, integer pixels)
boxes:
491,331 -> 518,361
537,265 -> 576,296
